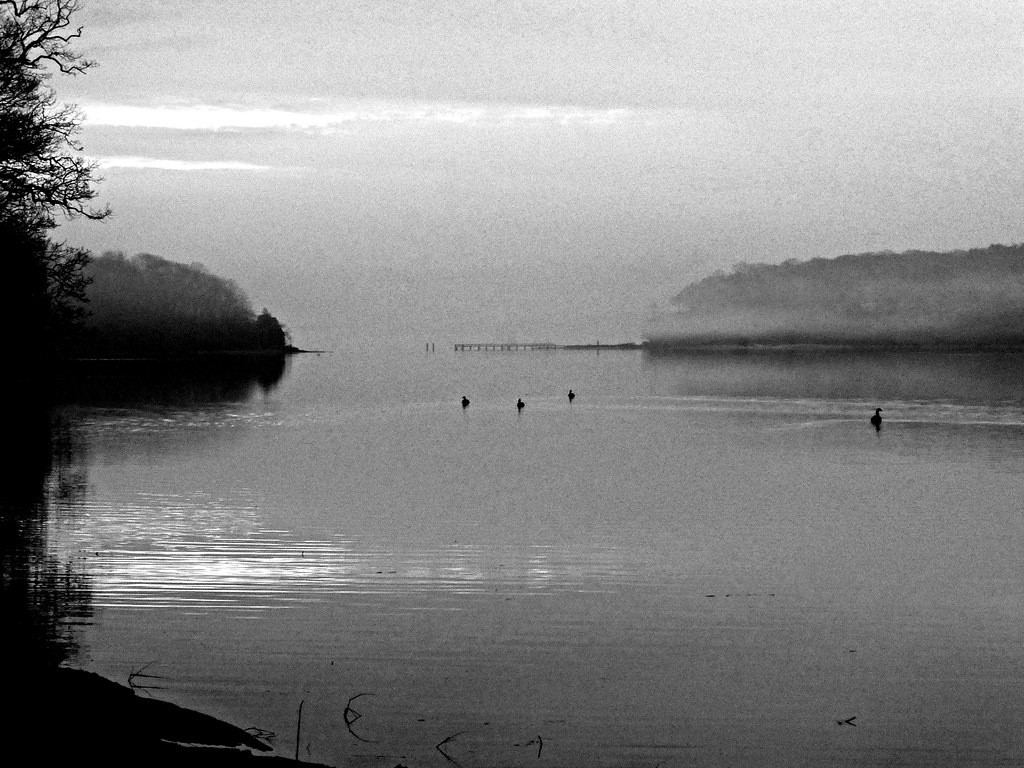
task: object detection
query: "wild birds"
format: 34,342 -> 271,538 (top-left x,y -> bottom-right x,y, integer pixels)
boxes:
462,396 -> 470,407
871,408 -> 882,425
516,399 -> 525,409
568,390 -> 575,400
